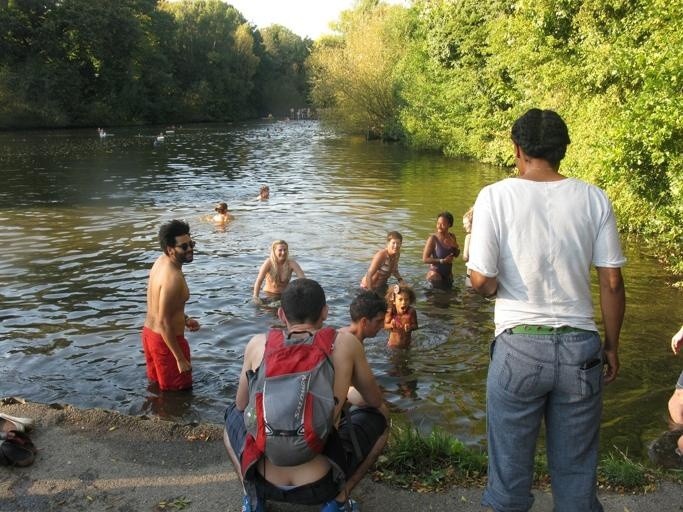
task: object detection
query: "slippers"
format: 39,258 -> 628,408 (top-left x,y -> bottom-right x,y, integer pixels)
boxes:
1,439 -> 35,467
1,430 -> 37,455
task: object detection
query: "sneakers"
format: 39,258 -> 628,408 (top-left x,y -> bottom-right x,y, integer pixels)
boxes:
1,417 -> 26,433
242,495 -> 262,512
0,413 -> 34,432
322,499 -> 359,511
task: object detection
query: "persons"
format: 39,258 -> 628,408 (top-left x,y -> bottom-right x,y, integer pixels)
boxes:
256,186 -> 269,199
142,219 -> 199,392
359,231 -> 405,291
467,108 -> 627,512
462,208 -> 475,288
212,203 -> 235,223
384,286 -> 419,349
341,291 -> 387,343
422,211 -> 461,285
667,328 -> 683,456
253,240 -> 305,305
223,279 -> 388,508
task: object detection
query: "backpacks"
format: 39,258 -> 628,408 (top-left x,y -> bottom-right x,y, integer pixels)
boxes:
244,327 -> 339,467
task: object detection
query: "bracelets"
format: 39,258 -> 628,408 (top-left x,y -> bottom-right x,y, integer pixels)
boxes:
185,315 -> 189,321
